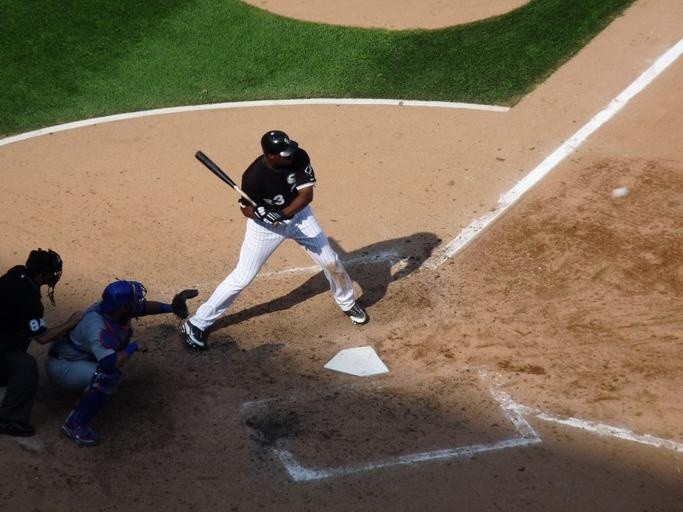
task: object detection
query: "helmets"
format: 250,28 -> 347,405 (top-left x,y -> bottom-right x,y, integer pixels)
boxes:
25,247 -> 62,307
261,130 -> 298,157
102,280 -> 147,321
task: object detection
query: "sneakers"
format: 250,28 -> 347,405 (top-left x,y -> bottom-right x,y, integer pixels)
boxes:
62,421 -> 95,445
181,320 -> 205,349
345,305 -> 368,325
0,422 -> 33,436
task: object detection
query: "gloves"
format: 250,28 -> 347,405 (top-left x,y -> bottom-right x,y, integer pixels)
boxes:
253,204 -> 266,219
263,211 -> 281,225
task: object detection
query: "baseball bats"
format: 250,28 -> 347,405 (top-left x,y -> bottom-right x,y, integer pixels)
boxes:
194,151 -> 278,227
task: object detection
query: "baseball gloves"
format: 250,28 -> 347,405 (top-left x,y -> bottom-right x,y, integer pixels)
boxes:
172,290 -> 198,319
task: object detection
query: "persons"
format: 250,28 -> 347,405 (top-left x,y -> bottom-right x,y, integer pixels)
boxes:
0,246 -> 84,439
181,129 -> 368,350
45,280 -> 197,449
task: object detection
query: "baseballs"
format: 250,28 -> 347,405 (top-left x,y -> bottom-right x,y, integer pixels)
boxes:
612,188 -> 629,199
